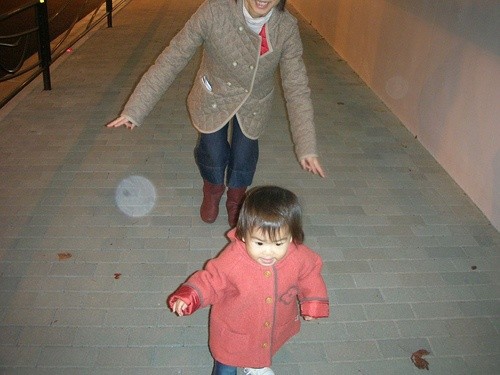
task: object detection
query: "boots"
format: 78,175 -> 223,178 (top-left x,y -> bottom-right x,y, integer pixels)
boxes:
199,177 -> 226,224
227,186 -> 247,229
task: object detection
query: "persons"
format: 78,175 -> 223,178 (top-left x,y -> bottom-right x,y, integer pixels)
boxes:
105,1 -> 327,231
167,187 -> 330,373
